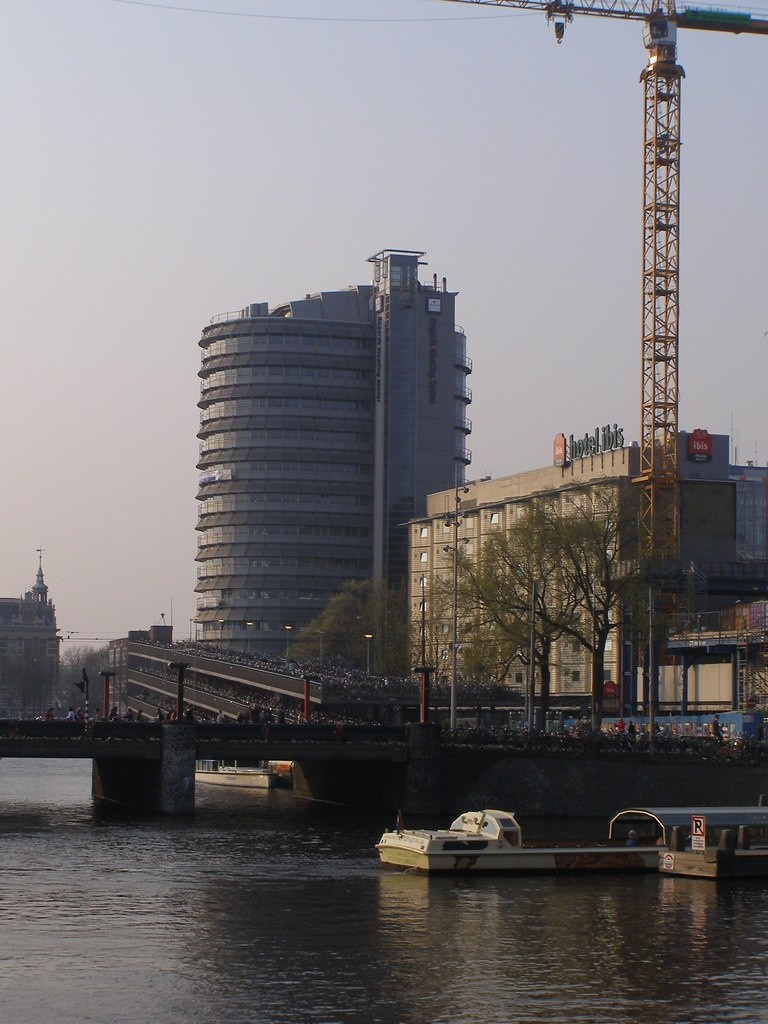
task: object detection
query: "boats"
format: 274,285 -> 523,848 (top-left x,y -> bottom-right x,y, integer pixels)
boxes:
375,804 -> 768,873
195,759 -> 279,789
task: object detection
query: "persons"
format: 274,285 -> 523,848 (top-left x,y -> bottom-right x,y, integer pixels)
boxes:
235,703 -> 287,724
95,708 -> 102,722
166,709 -> 177,722
109,706 -> 117,720
709,714 -> 723,743
187,710 -> 195,720
628,721 -> 635,734
155,709 -> 165,722
136,710 -> 143,721
66,707 -> 84,721
45,707 -> 54,721
123,708 -> 134,721
617,719 -> 625,731
575,716 -> 589,728
625,830 -> 641,847
217,710 -> 226,724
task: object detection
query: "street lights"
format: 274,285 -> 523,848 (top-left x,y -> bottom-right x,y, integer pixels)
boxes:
285,625 -> 292,660
218,620 -> 224,650
246,622 -> 252,654
365,634 -> 373,673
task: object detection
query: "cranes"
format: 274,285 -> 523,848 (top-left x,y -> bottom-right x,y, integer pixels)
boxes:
453,0 -> 768,566
